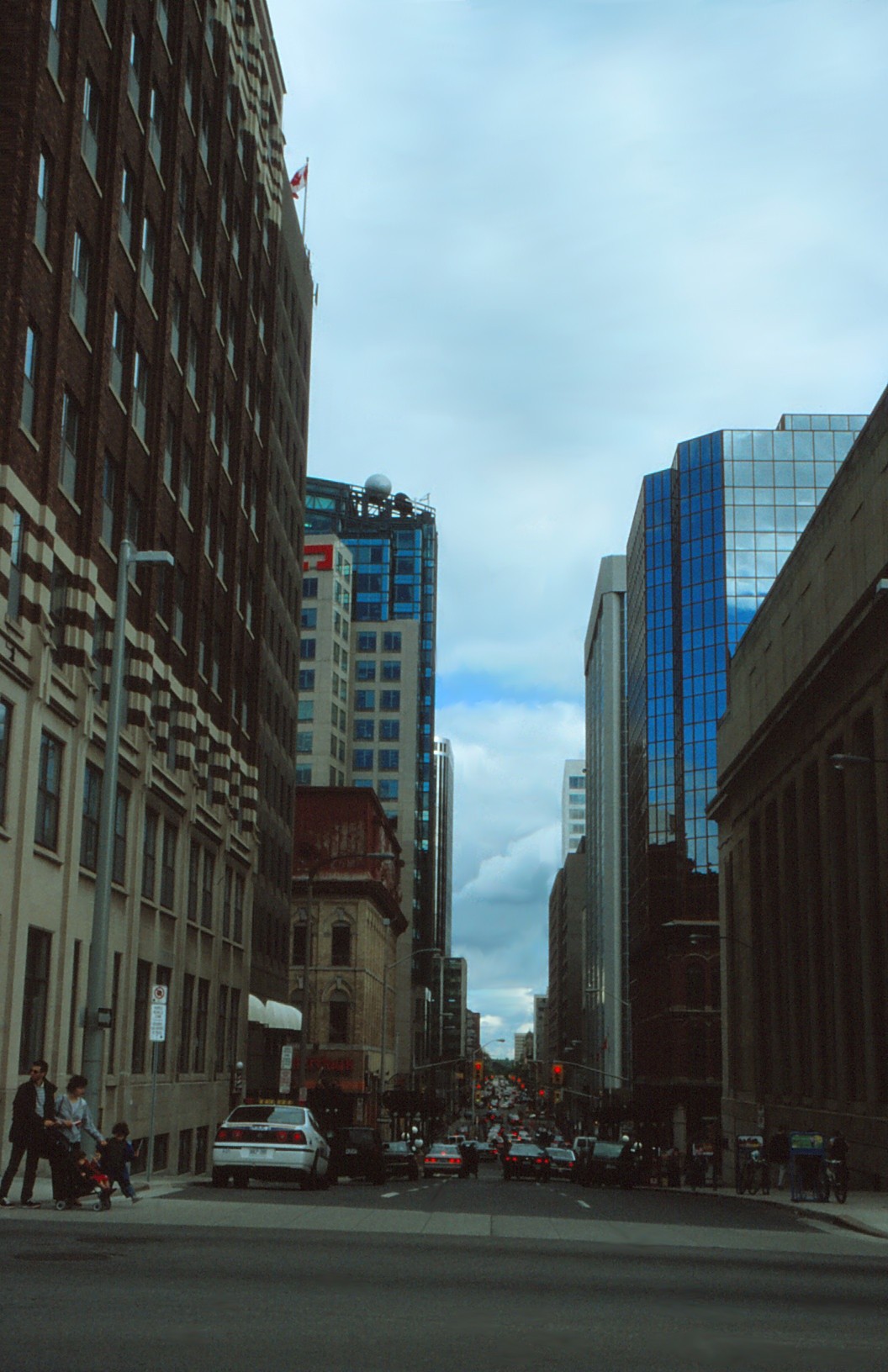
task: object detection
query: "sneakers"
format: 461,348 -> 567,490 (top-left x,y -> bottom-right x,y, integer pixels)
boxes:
19,1199 -> 41,1210
1,1197 -> 15,1210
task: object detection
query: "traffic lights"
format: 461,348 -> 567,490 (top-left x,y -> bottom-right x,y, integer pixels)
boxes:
473,1060 -> 483,1081
552,1064 -> 564,1085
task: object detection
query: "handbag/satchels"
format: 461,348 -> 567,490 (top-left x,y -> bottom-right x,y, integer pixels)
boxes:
39,1127 -> 69,1159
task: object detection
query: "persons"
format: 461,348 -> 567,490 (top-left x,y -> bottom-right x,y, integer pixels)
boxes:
668,1148 -> 681,1189
771,1124 -> 791,1188
98,1121 -> 142,1203
826,1130 -> 849,1184
51,1076 -> 107,1206
693,1156 -> 709,1186
0,1059 -> 59,1209
74,1150 -> 117,1195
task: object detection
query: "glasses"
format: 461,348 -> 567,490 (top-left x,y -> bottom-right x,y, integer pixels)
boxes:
29,1068 -> 44,1075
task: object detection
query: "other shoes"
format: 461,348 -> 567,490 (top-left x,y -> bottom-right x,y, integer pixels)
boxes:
108,1186 -> 117,1195
101,1187 -> 112,1196
71,1197 -> 82,1209
131,1196 -> 144,1204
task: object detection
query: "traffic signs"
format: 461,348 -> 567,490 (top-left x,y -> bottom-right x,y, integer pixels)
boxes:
149,1004 -> 167,1043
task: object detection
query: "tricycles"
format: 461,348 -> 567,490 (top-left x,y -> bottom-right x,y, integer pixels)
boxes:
687,1140 -> 722,1190
789,1131 -> 851,1202
738,1134 -> 771,1196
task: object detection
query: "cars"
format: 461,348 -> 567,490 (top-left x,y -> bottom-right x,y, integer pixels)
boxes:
212,1104 -> 332,1190
421,1072 -> 685,1191
324,1126 -> 380,1183
381,1142 -> 419,1181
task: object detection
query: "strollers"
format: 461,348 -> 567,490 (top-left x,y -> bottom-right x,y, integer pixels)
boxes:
29,1123 -> 112,1211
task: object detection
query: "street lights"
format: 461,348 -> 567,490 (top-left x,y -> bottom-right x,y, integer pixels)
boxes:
297,852 -> 396,1106
471,1039 -> 505,1139
409,1057 -> 470,1140
571,1038 -> 604,1110
688,933 -> 766,1135
376,947 -> 441,1117
76,539 -> 176,1157
585,988 -> 632,1112
